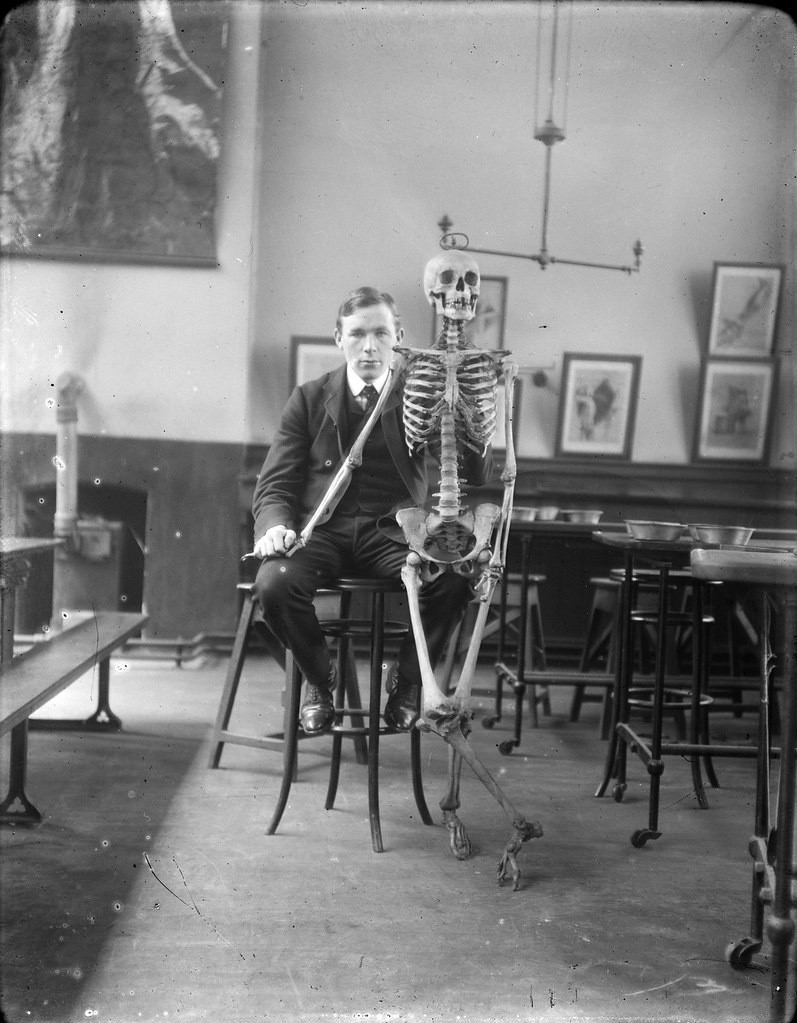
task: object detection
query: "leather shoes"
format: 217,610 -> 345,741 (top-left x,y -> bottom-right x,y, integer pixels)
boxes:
384,659 -> 421,733
297,658 -> 338,735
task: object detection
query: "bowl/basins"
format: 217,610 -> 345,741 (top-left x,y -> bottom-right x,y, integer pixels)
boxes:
624,518 -> 755,547
497,504 -> 605,525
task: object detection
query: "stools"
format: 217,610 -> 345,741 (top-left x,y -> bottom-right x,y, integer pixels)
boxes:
205,582 -> 368,781
265,572 -> 434,854
440,568 -> 746,811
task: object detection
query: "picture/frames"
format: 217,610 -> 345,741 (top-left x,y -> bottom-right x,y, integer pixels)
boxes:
705,260 -> 787,358
690,353 -> 781,467
286,335 -> 346,400
554,350 -> 642,463
492,378 -> 523,457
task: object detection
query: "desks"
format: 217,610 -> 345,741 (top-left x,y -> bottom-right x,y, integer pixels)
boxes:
690,546 -> 797,1023
0,538 -> 67,664
482,517 -> 797,758
589,531 -> 797,848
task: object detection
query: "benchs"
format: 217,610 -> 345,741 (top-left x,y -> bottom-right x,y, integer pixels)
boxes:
0,611 -> 150,825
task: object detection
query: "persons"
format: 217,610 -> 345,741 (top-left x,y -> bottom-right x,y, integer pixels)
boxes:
252,287 -> 494,736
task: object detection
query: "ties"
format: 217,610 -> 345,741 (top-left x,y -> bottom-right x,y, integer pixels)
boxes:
358,383 -> 380,413
